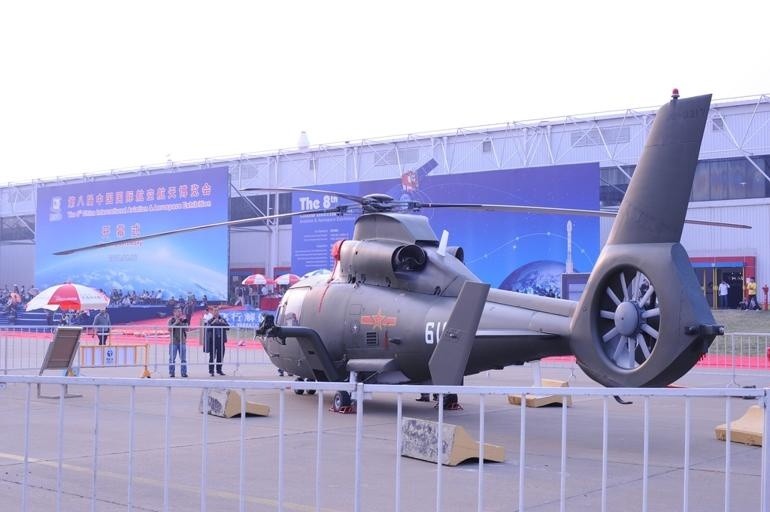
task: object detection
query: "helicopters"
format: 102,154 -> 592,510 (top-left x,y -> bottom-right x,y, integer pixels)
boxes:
49,86 -> 754,416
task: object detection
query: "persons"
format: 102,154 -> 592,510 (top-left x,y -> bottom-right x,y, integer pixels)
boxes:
207,305 -> 226,376
99,287 -> 209,331
93,307 -> 111,345
0,283 -> 90,327
231,283 -> 287,307
167,305 -> 189,378
707,282 -> 713,306
718,280 -> 730,309
744,276 -> 759,312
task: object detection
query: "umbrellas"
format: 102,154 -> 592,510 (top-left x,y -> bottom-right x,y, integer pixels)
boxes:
275,273 -> 300,286
24,281 -> 110,325
242,273 -> 275,291
